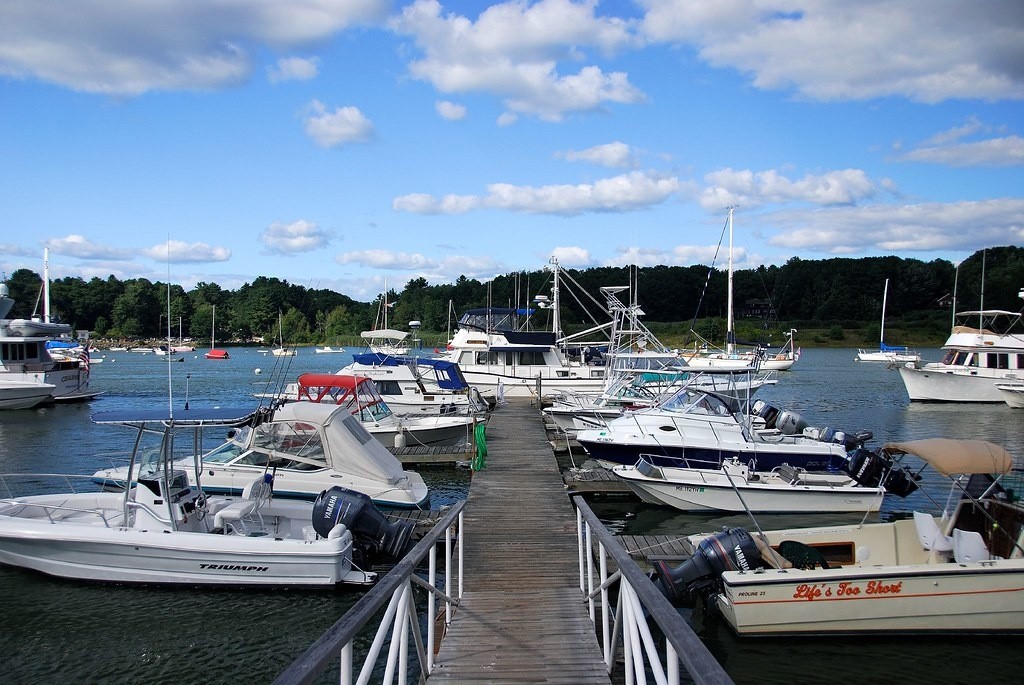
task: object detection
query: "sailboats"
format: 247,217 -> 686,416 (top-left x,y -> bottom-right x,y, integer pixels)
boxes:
599,203 -> 801,372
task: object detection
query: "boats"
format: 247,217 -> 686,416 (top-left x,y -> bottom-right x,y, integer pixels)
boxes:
361,328 -> 413,355
0,407 -> 416,591
132,402 -> 431,513
895,248 -> 1024,400
576,387 -> 873,470
0,247 -> 106,410
284,327 -> 779,401
857,278 -> 920,365
296,373 -> 486,446
109,301 -> 347,360
611,447 -> 921,516
652,436 -> 1023,633
250,351 -> 489,417
416,358 -> 506,411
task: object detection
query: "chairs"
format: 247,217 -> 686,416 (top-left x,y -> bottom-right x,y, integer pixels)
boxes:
952,528 -> 1003,564
913,511 -> 954,564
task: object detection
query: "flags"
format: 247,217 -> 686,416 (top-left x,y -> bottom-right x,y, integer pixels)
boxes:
78,345 -> 90,375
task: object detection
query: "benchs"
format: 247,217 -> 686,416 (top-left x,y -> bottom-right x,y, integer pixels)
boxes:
762,463 -> 800,487
216,460 -> 279,536
